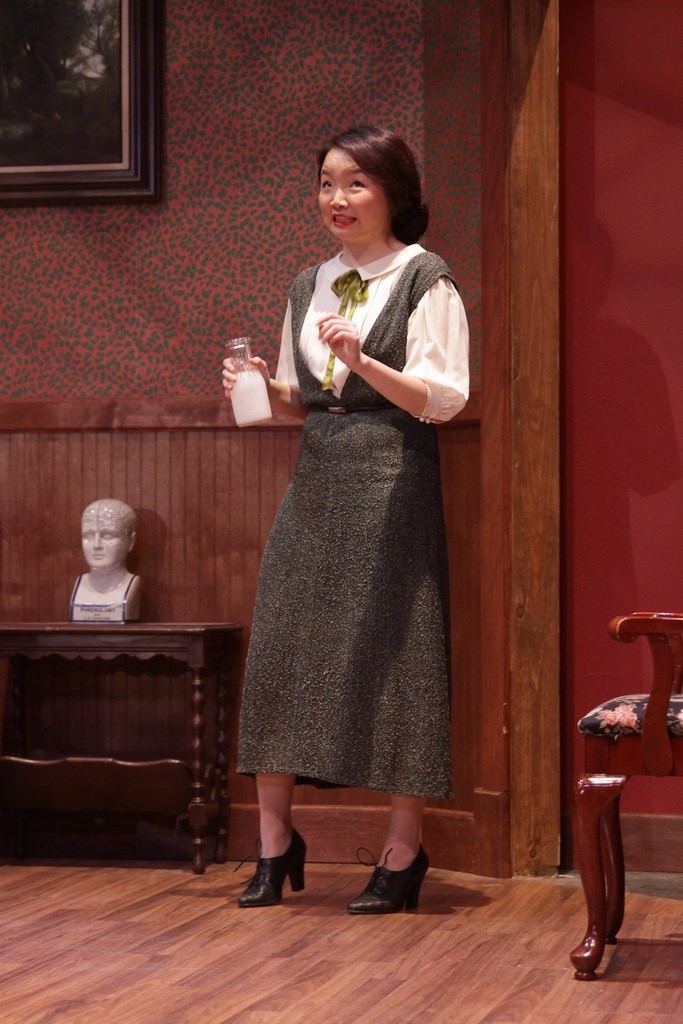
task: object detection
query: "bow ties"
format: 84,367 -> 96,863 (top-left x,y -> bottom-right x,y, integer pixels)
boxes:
323,268 -> 369,389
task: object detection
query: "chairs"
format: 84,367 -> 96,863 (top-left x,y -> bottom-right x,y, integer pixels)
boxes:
568,612 -> 683,980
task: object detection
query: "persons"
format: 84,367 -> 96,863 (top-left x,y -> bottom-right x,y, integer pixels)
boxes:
221,120 -> 471,914
66,498 -> 144,623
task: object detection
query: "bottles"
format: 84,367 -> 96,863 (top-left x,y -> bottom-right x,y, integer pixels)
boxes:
225,338 -> 272,427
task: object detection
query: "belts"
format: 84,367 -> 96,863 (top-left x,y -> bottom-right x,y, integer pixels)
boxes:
317,404 -> 397,413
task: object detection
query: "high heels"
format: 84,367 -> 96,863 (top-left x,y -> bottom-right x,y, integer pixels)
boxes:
347,843 -> 430,914
234,828 -> 307,908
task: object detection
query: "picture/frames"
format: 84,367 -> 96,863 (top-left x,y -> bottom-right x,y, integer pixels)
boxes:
0,0 -> 164,206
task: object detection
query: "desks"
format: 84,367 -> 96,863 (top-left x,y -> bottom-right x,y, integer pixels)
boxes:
0,621 -> 243,876
